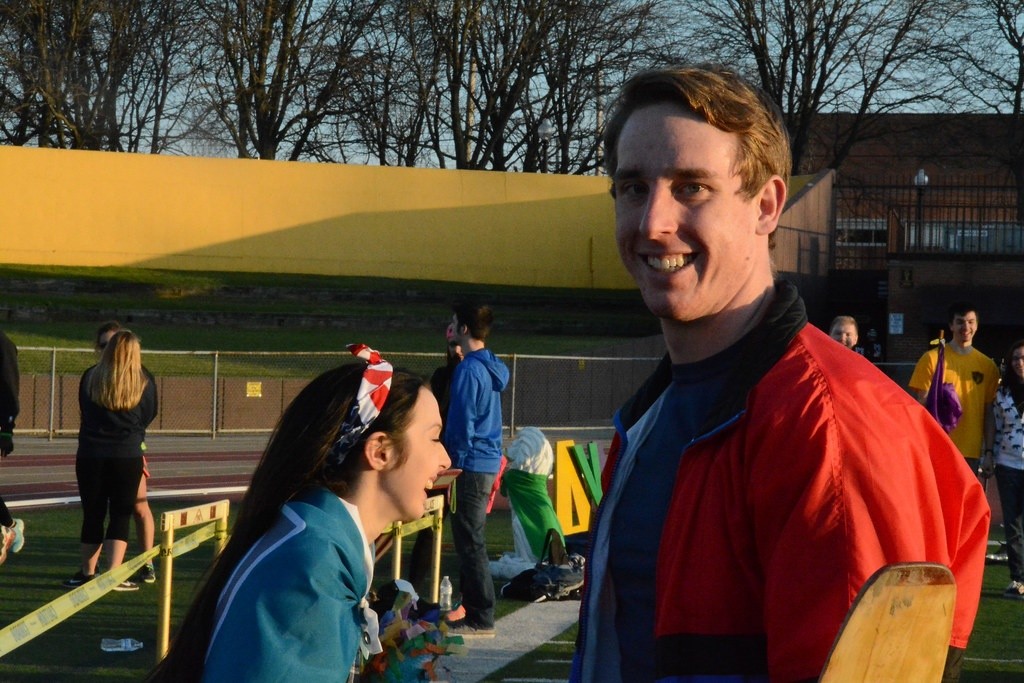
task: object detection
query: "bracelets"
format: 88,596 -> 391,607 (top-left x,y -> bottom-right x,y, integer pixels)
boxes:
984,449 -> 993,456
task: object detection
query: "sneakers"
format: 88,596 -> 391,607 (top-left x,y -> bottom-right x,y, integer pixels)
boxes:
446,618 -> 495,640
61,567 -> 101,588
0,525 -> 16,564
1004,580 -> 1024,599
9,518 -> 24,553
113,579 -> 140,591
142,564 -> 156,582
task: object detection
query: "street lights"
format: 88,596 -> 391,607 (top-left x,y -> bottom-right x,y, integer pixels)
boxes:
913,168 -> 930,250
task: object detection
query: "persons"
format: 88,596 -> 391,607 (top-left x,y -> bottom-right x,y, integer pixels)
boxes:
829,316 -> 858,350
96,321 -> 159,583
908,300 -> 1000,476
445,305 -> 510,633
0,330 -> 24,566
571,64 -> 991,683
140,344 -> 452,683
407,324 -> 466,621
57,331 -> 140,591
993,340 -> 1024,599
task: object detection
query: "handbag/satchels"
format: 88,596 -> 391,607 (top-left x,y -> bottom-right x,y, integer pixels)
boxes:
500,529 -> 585,602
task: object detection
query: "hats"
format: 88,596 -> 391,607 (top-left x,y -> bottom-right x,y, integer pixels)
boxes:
447,324 -> 457,344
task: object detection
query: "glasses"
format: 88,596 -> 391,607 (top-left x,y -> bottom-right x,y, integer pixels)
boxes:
98,341 -> 108,349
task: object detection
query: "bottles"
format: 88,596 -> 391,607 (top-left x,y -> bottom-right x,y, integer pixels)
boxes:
439,576 -> 452,609
100,638 -> 143,651
